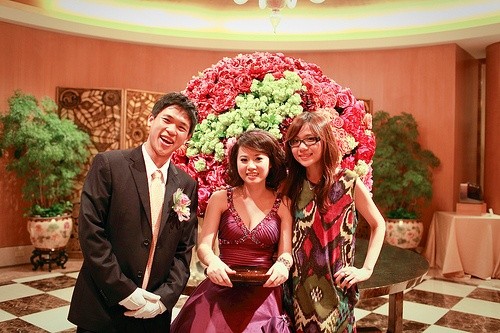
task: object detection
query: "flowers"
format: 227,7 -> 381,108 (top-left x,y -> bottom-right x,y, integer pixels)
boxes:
172,187 -> 192,222
172,49 -> 378,228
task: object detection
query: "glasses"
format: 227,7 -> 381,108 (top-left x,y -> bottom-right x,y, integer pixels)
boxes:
288,136 -> 321,147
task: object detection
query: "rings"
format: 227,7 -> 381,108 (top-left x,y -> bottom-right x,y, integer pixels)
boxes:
344,272 -> 347,276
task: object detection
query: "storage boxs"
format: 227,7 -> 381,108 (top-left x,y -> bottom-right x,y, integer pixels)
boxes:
456,202 -> 487,215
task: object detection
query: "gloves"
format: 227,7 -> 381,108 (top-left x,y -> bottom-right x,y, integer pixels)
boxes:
119,287 -> 162,320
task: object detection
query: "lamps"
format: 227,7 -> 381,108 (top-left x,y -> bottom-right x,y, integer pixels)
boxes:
234,0 -> 326,34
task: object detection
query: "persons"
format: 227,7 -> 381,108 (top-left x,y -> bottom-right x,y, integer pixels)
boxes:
170,130 -> 293,333
67,92 -> 198,333
278,113 -> 387,333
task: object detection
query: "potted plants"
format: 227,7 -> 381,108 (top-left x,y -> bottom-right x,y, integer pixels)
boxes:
371,109 -> 441,250
0,88 -> 94,251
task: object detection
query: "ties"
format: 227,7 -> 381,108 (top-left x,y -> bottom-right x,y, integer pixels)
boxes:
147,170 -> 167,274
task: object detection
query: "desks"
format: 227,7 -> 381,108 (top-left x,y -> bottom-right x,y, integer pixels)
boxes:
427,210 -> 500,282
355,237 -> 430,333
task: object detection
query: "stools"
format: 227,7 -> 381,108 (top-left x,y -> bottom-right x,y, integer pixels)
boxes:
29,249 -> 68,272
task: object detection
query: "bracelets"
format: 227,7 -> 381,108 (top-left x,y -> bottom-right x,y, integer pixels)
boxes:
278,257 -> 291,269
